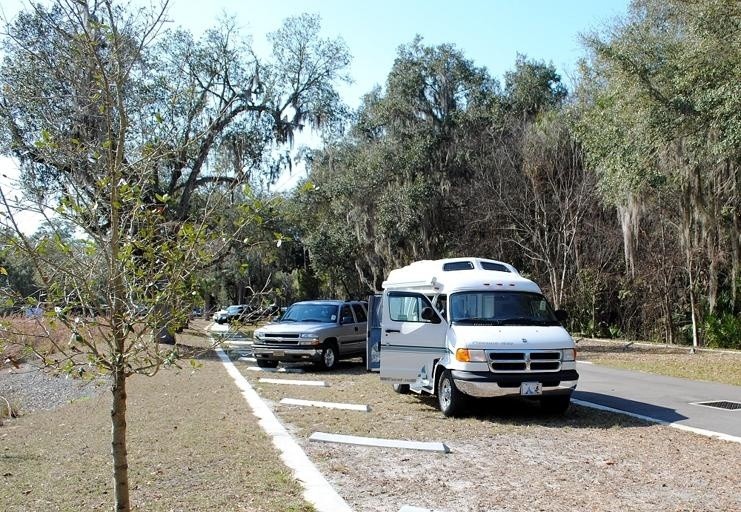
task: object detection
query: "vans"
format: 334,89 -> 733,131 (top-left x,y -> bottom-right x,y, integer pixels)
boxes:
367,256 -> 580,416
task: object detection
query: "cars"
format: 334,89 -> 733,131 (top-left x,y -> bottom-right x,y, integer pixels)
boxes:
252,300 -> 369,371
213,305 -> 258,324
